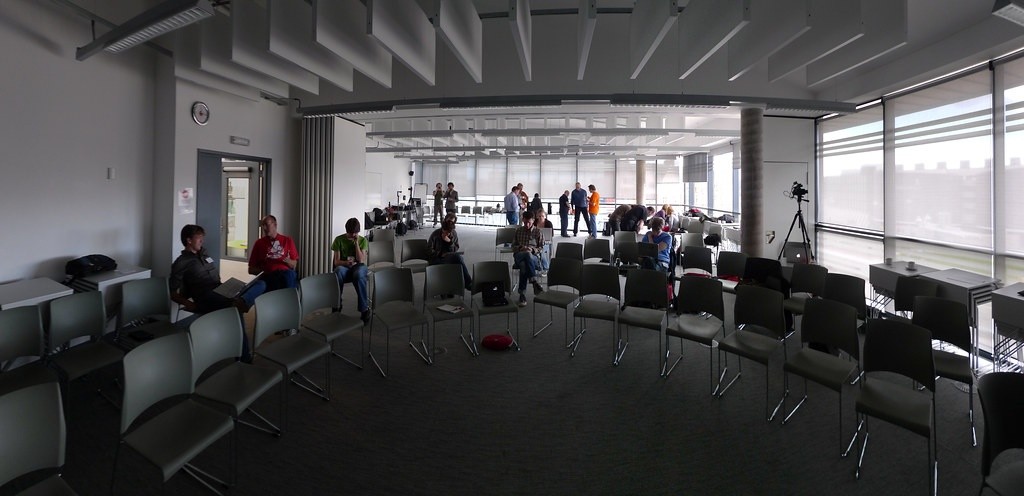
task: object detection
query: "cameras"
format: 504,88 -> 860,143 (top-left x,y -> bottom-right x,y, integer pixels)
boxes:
792,188 -> 808,195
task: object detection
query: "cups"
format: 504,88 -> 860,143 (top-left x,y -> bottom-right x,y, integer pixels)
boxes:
886,258 -> 892,265
722,221 -> 726,224
718,220 -> 721,224
908,261 -> 915,269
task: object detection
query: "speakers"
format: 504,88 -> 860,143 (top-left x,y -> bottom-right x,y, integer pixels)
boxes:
409,171 -> 414,176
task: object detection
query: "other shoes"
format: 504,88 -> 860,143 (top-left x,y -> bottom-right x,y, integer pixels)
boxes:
361,309 -> 370,325
534,284 -> 545,296
519,294 -> 528,307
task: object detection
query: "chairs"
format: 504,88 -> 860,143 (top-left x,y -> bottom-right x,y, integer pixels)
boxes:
532,257 -> 587,348
614,268 -> 670,377
716,251 -> 749,279
976,371 -> 1024,496
555,243 -> 583,260
613,241 -> 637,264
496,228 -> 517,266
636,242 -> 659,266
825,272 -> 867,328
782,297 -> 864,458
614,231 -> 637,243
662,276 -> 728,396
682,217 -> 689,230
400,238 -> 431,272
470,260 -> 520,356
570,264 -> 621,366
708,222 -> 724,265
420,263 -> 476,364
584,239 -> 611,264
432,206 -> 522,227
687,219 -> 705,233
734,257 -> 794,334
783,264 -> 829,331
682,246 -> 713,279
725,228 -> 741,253
855,319 -> 939,496
910,295 -> 978,447
680,233 -> 704,267
539,227 -> 555,259
0,204 -> 430,496
719,284 -> 790,422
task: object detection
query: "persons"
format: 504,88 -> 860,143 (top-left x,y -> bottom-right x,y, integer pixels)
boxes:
331,218 -> 371,323
511,211 -> 545,307
168,225 -> 266,365
636,216 -> 674,310
425,212 -> 473,291
432,182 -> 701,239
248,215 -> 300,336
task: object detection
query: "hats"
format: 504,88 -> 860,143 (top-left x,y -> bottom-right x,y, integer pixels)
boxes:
651,217 -> 664,228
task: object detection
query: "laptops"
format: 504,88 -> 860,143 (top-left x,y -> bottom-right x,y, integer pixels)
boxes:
213,271 -> 264,300
638,241 -> 660,266
785,242 -> 812,264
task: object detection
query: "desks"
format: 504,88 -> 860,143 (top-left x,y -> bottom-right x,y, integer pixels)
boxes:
79,260 -> 152,337
705,220 -> 740,251
387,209 -> 416,223
0,276 -> 73,351
868,262 -> 1024,378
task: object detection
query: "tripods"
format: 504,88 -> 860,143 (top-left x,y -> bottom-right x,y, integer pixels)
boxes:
778,196 -> 815,265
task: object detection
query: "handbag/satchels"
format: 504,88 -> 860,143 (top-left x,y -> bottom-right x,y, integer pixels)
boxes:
365,205 -> 418,241
62,254 -> 117,286
482,334 -> 514,352
480,280 -> 510,308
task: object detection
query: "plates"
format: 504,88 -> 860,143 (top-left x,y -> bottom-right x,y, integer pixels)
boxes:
906,266 -> 917,270
